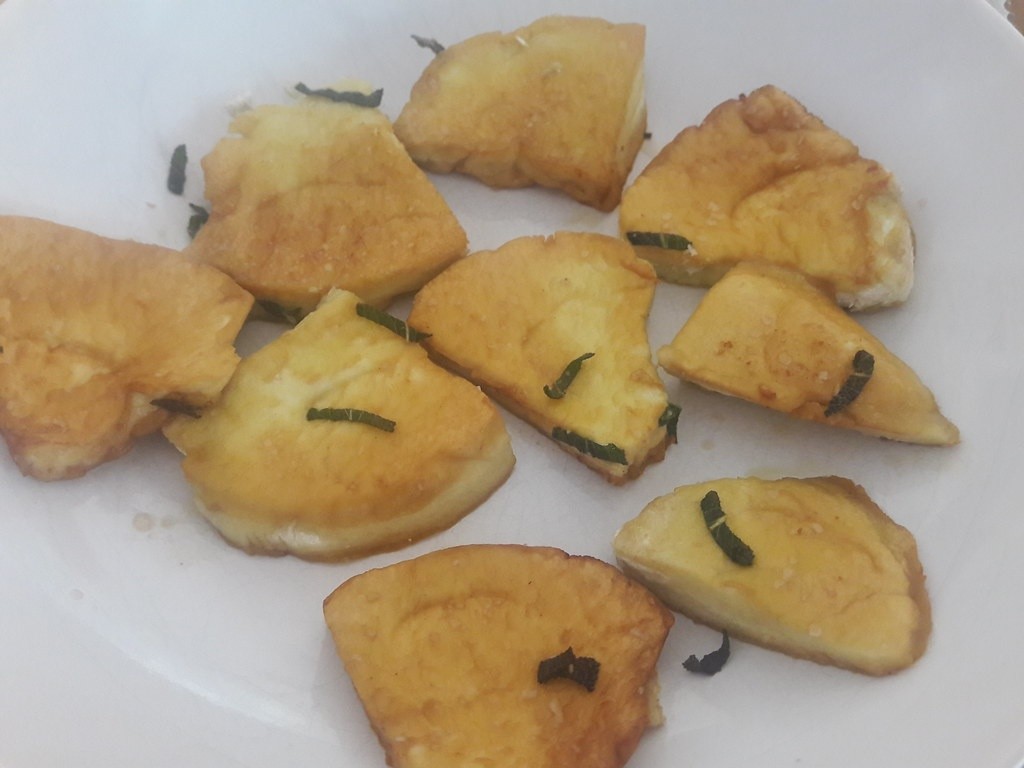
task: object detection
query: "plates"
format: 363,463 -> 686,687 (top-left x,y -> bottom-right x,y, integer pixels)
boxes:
3,2 -> 1023,768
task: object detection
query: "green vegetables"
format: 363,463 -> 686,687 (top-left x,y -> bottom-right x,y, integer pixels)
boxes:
146,39 -> 877,692
